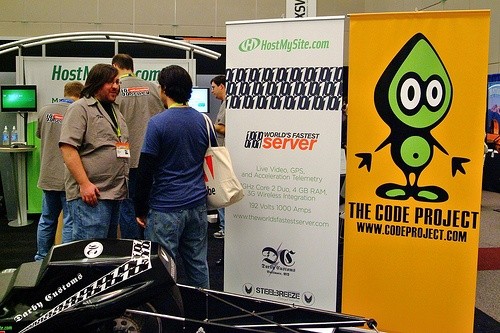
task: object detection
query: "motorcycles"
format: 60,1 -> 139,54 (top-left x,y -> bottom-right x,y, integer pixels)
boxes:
0,237 -> 184,333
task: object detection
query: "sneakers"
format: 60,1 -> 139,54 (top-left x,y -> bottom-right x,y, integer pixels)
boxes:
213,230 -> 224,238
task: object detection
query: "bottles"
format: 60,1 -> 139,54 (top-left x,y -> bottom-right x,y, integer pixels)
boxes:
11,126 -> 18,147
2,126 -> 10,147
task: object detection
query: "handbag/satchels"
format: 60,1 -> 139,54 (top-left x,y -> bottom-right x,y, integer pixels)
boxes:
201,113 -> 245,210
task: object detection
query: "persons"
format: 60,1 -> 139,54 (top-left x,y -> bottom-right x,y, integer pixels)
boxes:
58,63 -> 130,243
124,150 -> 128,154
34,81 -> 86,261
111,54 -> 166,241
209,75 -> 226,238
132,65 -> 217,290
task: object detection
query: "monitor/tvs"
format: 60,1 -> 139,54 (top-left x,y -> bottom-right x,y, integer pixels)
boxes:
189,87 -> 210,113
0,85 -> 37,113
